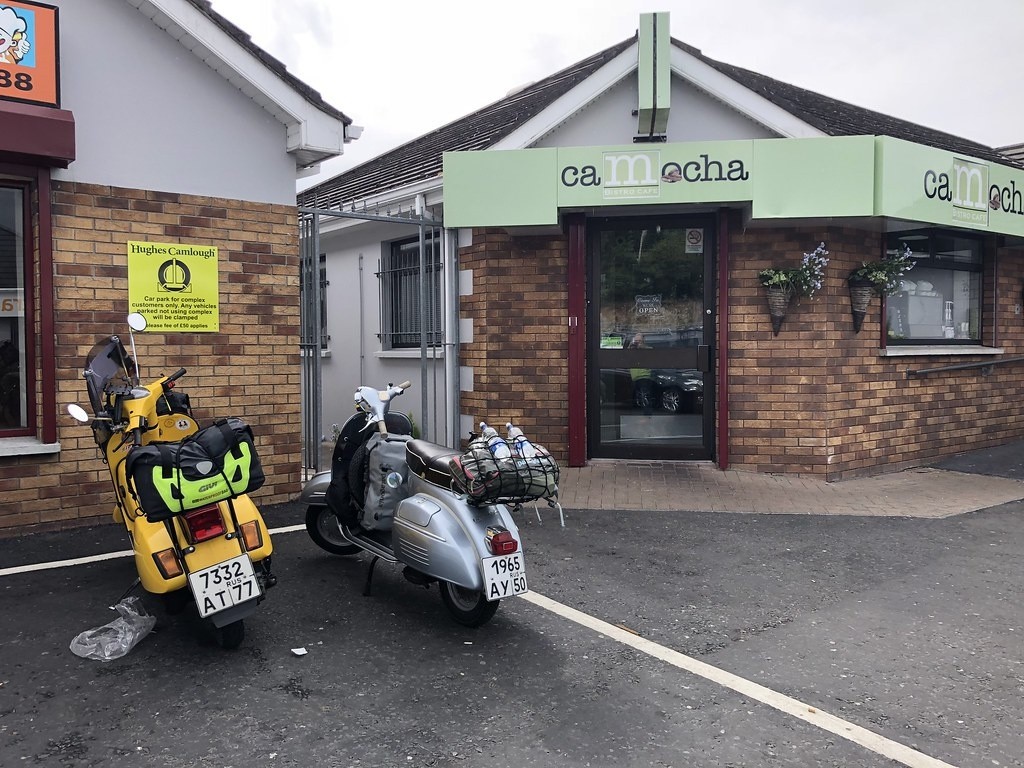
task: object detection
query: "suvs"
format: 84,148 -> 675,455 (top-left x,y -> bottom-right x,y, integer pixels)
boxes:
600,327 -> 705,411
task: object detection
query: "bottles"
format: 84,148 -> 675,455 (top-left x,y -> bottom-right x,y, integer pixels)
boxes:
506,422 -> 536,461
480,422 -> 512,462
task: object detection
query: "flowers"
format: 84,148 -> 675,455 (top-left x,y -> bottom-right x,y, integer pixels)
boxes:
850,242 -> 917,296
760,239 -> 833,304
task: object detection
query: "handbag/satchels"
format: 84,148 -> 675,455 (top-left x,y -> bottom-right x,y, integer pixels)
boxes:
127,416 -> 268,522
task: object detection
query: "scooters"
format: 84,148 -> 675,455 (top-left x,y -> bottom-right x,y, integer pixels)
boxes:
300,380 -> 563,628
66,313 -> 277,650
